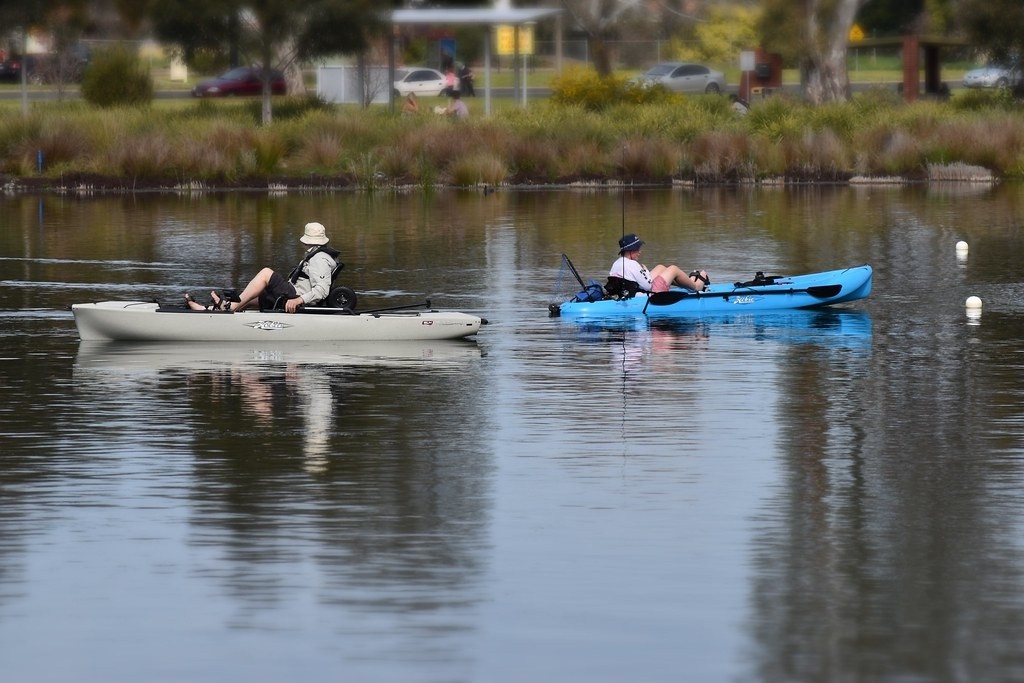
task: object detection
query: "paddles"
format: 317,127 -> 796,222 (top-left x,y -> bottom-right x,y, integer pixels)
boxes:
353,298 -> 431,314
648,284 -> 842,306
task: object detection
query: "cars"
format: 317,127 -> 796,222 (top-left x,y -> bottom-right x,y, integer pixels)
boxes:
626,63 -> 726,93
962,61 -> 1023,90
1,51 -> 85,87
192,65 -> 288,99
393,66 -> 451,96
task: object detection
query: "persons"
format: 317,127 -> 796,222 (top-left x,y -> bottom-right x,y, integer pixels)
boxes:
448,92 -> 468,118
402,92 -> 418,112
184,222 -> 339,313
611,234 -> 707,292
457,64 -> 475,97
728,94 -> 748,112
445,68 -> 455,90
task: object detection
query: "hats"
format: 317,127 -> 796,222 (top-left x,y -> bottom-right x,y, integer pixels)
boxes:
300,222 -> 330,245
617,233 -> 645,255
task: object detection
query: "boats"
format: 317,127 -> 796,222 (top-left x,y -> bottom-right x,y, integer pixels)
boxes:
550,264 -> 873,321
71,298 -> 482,341
